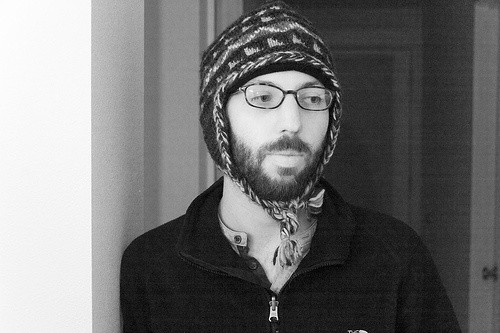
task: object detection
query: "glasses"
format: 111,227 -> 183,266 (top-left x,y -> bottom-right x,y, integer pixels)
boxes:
227,81 -> 340,113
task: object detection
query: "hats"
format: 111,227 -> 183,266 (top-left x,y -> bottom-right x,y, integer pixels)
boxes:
200,1 -> 343,271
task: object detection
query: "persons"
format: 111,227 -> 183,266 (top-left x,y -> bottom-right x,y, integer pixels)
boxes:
119,0 -> 464,333
